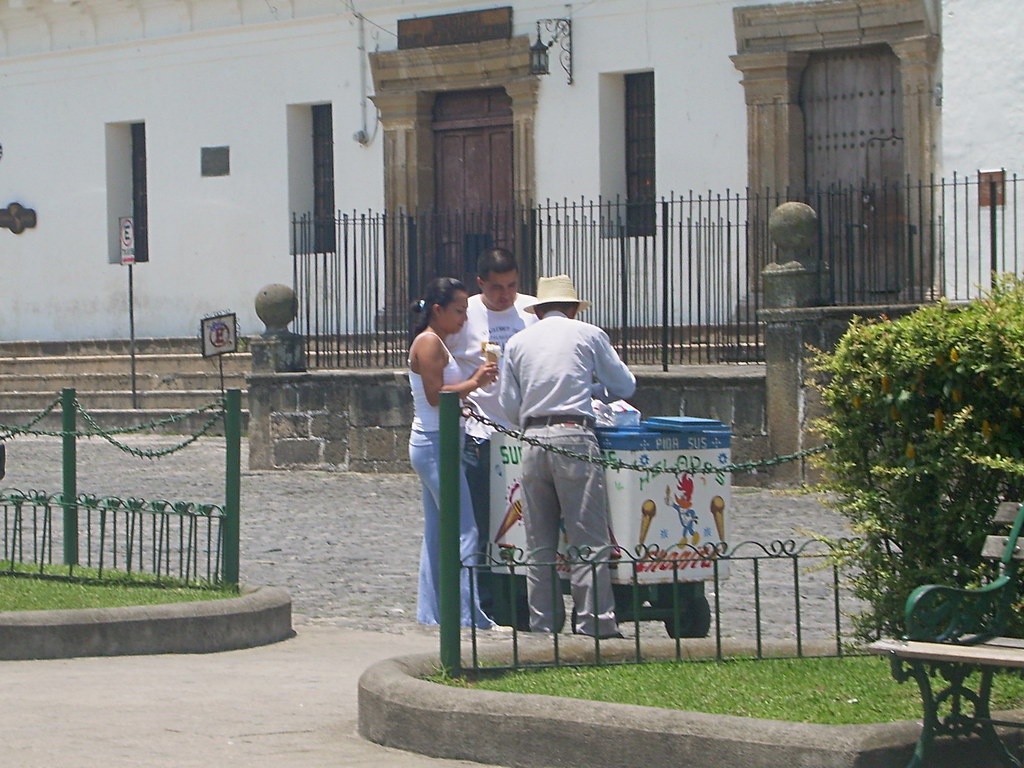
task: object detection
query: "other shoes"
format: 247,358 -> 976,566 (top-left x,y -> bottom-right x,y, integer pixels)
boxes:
486,623 -> 513,634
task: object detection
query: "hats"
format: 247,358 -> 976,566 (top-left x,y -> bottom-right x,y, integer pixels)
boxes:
523,274 -> 591,314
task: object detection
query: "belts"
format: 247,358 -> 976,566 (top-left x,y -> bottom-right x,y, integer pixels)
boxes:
527,415 -> 594,428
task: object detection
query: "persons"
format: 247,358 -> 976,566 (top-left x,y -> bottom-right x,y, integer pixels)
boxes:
407,247 -> 637,641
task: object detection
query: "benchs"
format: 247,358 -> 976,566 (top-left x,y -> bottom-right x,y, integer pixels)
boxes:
863,501 -> 1024,768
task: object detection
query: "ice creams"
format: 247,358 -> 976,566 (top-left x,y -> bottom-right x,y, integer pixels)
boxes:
485,342 -> 502,363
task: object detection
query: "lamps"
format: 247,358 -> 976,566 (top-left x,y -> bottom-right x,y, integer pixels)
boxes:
527,15 -> 574,87
